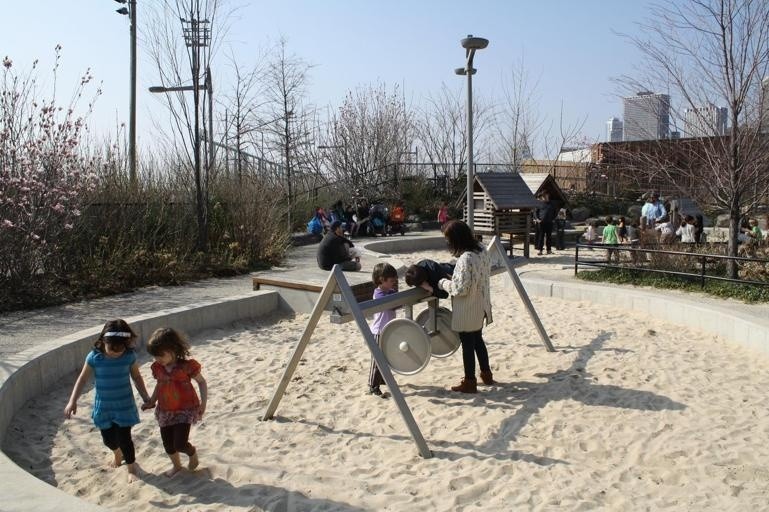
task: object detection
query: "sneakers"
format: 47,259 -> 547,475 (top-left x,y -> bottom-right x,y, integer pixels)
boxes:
370,386 -> 385,398
452,380 -> 477,392
481,375 -> 492,384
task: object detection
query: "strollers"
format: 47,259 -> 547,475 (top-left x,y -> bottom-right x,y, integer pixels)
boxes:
366,201 -> 406,238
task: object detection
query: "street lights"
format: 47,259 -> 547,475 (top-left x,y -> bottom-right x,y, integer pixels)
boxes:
317,143 -> 350,192
455,32 -> 490,234
115,0 -> 140,181
148,82 -> 218,222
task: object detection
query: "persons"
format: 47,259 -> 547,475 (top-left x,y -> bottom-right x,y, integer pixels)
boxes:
438,220 -> 493,392
64,319 -> 155,483
585,189 -> 702,267
534,190 -> 566,255
317,221 -> 361,271
141,327 -> 207,478
307,198 -> 407,243
437,201 -> 448,232
367,262 -> 403,399
738,206 -> 763,247
406,259 -> 456,299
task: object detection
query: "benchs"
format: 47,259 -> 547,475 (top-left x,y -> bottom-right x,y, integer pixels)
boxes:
530,220 -> 588,247
292,231 -> 311,246
252,265 -> 374,309
